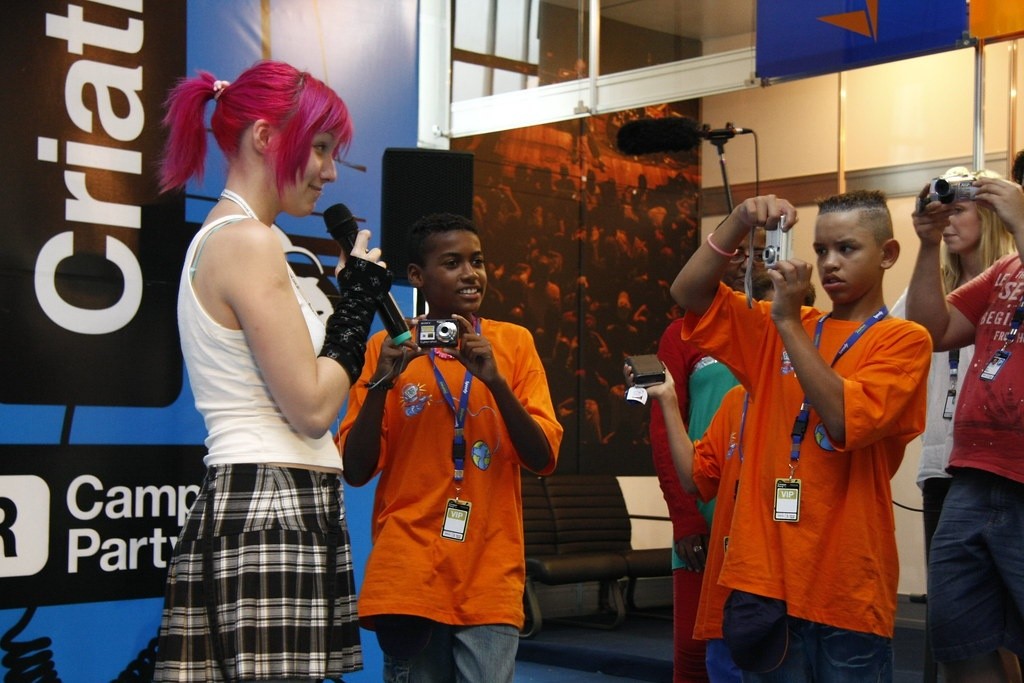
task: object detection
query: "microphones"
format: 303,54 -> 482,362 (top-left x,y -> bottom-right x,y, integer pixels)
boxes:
616,115 -> 748,158
321,202 -> 412,346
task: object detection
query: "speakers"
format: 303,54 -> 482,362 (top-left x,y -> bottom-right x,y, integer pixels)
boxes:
381,147 -> 474,287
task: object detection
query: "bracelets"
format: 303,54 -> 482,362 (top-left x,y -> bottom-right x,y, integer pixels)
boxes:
363,379 -> 395,392
705,233 -> 738,256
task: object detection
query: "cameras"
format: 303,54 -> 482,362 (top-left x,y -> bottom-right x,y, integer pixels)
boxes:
623,354 -> 667,390
417,317 -> 460,347
929,175 -> 981,205
761,213 -> 793,269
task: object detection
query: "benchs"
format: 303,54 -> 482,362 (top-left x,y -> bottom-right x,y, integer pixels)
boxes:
517,476 -> 675,640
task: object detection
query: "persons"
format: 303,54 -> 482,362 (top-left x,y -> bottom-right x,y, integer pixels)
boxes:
648,212 -> 818,683
333,210 -> 564,682
668,190 -> 933,681
149,59 -> 393,683
889,150 -> 1023,683
